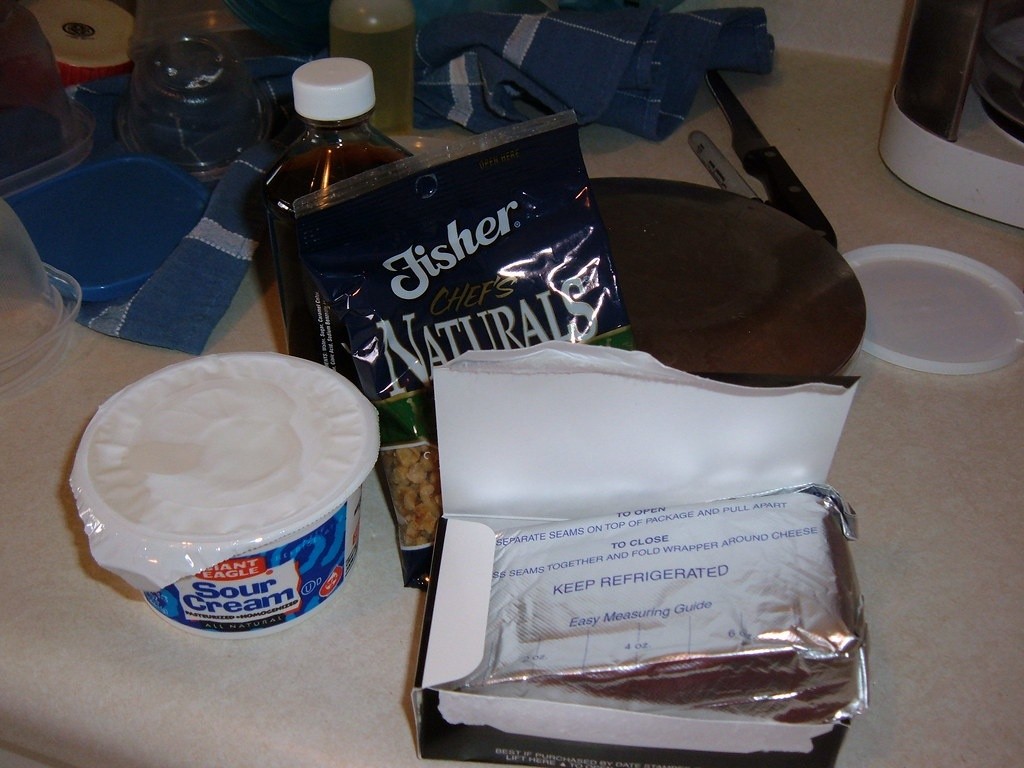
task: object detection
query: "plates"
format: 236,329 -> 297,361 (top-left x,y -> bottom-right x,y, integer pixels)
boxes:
586,177 -> 867,389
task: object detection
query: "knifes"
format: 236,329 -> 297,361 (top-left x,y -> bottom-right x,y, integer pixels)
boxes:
701,68 -> 837,252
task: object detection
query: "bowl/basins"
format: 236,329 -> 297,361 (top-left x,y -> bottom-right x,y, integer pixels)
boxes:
117,32 -> 273,182
0,190 -> 81,400
0,0 -> 97,198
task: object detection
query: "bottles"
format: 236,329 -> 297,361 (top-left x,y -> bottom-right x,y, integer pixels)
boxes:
254,58 -> 417,362
329,0 -> 415,136
